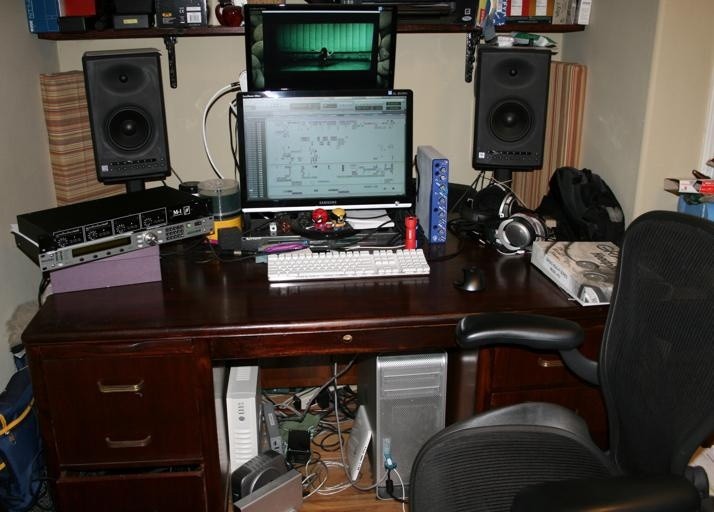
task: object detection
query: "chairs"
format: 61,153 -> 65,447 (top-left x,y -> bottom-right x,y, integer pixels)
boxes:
408,209 -> 714,511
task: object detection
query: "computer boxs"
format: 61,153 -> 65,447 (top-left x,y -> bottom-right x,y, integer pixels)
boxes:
357,353 -> 447,496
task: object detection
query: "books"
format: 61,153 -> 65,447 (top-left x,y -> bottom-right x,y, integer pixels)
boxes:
475,0 -> 592,26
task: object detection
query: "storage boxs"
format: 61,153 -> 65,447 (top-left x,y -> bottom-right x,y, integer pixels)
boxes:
50,245 -> 162,293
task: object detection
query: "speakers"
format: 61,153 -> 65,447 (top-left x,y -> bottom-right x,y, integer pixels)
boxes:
473,44 -> 551,170
83,48 -> 171,184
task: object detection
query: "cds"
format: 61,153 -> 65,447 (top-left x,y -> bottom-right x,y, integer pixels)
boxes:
197,180 -> 237,215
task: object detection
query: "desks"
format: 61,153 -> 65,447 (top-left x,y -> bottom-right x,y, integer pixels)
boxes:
21,210 -> 611,512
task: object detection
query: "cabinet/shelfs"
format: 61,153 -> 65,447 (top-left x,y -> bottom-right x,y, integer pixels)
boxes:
24,1 -> 592,88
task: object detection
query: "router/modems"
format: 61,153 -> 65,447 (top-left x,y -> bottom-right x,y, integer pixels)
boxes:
345,210 -> 396,230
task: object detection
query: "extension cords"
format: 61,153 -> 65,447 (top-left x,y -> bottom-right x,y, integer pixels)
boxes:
296,384 -> 323,410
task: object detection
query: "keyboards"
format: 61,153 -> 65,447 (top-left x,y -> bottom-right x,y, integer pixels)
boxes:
267,247 -> 432,283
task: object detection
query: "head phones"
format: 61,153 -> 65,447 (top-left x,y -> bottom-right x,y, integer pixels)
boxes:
451,212 -> 544,255
463,183 -> 517,221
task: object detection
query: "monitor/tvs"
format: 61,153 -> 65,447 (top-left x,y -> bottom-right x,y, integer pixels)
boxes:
245,5 -> 397,96
237,91 -> 412,239
